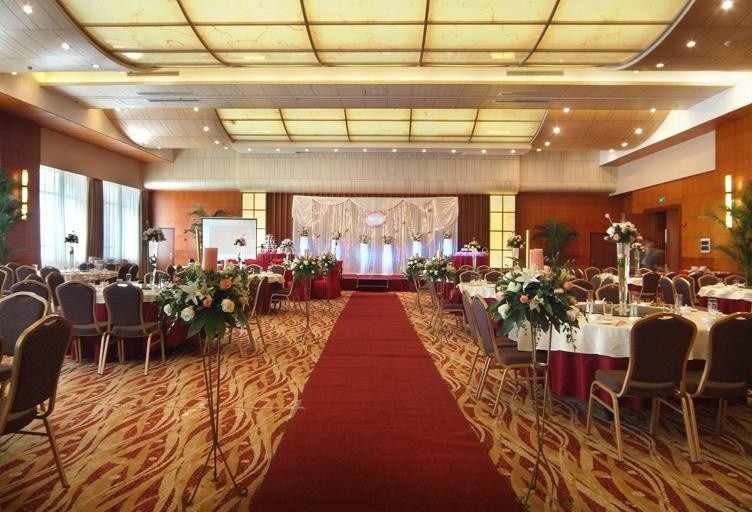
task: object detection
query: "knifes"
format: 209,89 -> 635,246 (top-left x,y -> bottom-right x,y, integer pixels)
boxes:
615,321 -> 626,326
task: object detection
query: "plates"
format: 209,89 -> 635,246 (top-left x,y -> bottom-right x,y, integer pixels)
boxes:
599,320 -> 614,325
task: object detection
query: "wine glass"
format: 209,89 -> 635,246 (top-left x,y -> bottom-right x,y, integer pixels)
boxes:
470,273 -> 482,286
585,287 -> 720,322
732,278 -> 741,291
87,273 -> 167,293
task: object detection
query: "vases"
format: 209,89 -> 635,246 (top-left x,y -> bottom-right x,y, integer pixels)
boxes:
69,248 -> 74,268
617,243 -> 630,314
512,248 -> 519,259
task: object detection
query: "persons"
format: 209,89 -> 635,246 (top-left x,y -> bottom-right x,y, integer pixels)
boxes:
633,238 -> 658,271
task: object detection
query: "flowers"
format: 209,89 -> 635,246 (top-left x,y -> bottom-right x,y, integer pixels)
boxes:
494,266 -> 590,356
423,256 -> 457,282
64,230 -> 79,246
404,255 -> 426,279
506,230 -> 526,249
234,237 -> 246,245
283,256 -> 328,279
299,227 -> 452,244
604,213 -> 643,279
320,251 -> 336,268
143,227 -> 166,243
156,262 -> 261,343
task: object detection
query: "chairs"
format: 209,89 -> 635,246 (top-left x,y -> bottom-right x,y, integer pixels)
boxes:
461,289 -> 528,388
1,314 -> 74,489
97,281 -> 166,376
54,279 -> 123,365
1,259 -> 66,316
469,293 -> 557,418
143,259 -> 300,313
414,267 -> 508,332
568,266 -> 751,313
585,313 -> 705,462
1,290 -> 49,412
77,259 -> 139,281
648,312 -> 752,437
201,275 -> 272,358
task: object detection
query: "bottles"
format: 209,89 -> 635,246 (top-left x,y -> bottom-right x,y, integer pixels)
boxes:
247,267 -> 254,278
267,268 -> 270,275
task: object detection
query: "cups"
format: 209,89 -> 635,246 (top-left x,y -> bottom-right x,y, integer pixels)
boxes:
498,277 -> 503,282
32,263 -> 38,270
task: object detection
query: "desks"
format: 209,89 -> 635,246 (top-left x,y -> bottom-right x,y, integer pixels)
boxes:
509,299 -> 746,413
